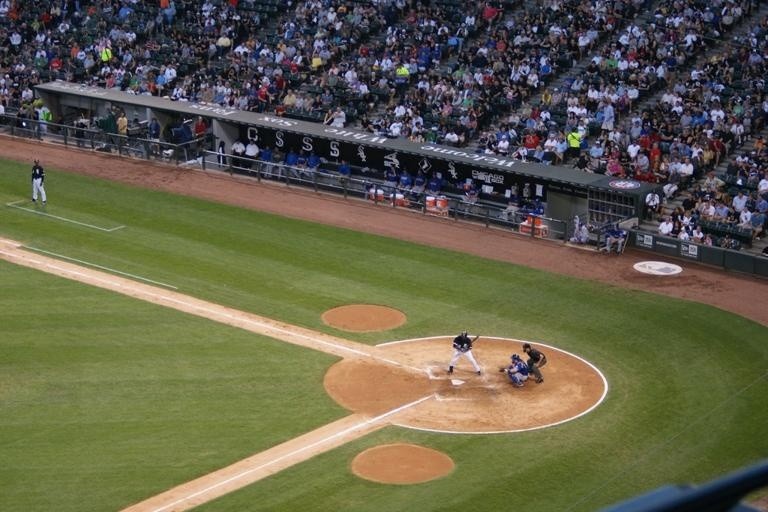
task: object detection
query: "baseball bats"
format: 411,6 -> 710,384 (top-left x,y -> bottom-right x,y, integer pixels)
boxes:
461,336 -> 478,352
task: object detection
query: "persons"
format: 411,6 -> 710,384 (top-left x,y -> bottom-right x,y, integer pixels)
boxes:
522,343 -> 547,383
448,329 -> 482,375
0,2 -> 768,254
499,353 -> 529,386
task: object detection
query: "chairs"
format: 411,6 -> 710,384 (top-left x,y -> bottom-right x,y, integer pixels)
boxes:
0,0 -> 768,255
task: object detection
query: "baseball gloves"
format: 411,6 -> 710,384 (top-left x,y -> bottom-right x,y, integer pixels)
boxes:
498,366 -> 505,372
